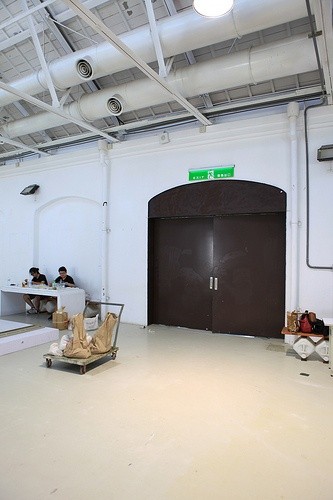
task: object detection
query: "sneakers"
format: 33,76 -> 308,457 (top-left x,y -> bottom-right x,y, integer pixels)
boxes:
27,307 -> 39,314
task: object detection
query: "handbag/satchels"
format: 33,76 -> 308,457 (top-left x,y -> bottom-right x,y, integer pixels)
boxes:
291,312 -> 312,333
307,313 -> 327,334
287,310 -> 298,333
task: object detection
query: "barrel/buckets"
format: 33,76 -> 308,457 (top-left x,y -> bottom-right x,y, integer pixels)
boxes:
52,311 -> 69,330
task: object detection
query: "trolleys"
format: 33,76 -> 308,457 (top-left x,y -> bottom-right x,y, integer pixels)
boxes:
44,301 -> 126,375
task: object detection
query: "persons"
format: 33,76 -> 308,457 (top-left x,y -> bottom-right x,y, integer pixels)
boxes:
23,267 -> 48,314
48,267 -> 77,322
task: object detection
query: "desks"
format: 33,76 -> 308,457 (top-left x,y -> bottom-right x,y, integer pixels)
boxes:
281,327 -> 329,363
0,285 -> 85,317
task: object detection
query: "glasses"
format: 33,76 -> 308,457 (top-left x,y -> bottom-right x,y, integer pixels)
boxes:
31,272 -> 35,275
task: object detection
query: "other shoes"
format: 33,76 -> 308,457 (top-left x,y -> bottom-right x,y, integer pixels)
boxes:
48,314 -> 52,320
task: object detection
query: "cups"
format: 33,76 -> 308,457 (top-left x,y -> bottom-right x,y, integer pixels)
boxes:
52,283 -> 56,290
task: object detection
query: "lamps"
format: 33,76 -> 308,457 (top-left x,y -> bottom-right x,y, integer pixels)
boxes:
20,184 -> 40,195
